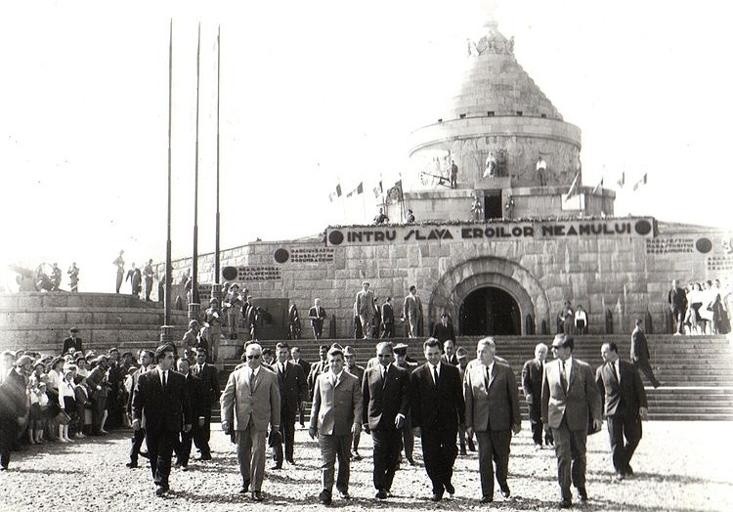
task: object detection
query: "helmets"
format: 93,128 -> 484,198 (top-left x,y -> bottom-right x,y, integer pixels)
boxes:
96,355 -> 109,364
14,356 -> 33,366
210,298 -> 218,305
189,320 -> 198,326
224,282 -> 249,293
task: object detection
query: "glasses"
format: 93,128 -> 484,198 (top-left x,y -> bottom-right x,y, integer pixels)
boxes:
248,355 -> 260,359
552,345 -> 566,350
379,355 -> 393,357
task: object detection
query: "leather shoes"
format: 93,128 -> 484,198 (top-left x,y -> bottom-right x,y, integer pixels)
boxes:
125,449 -> 151,468
446,484 -> 455,495
479,495 -> 493,503
339,491 -> 349,499
375,487 -> 392,499
460,442 -> 475,455
579,492 -> 586,502
501,486 -> 509,497
318,490 -> 332,504
560,497 -> 571,508
616,465 -> 633,481
349,451 -> 360,462
536,442 -> 554,450
155,484 -> 170,496
239,480 -> 263,501
172,450 -> 212,467
432,492 -> 443,502
396,457 -> 415,471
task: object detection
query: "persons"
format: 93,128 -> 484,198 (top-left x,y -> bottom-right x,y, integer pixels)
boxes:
595,341 -> 648,481
540,333 -> 604,508
16,261 -> 79,292
1,327 -> 217,494
630,319 -> 662,389
181,282 -> 266,365
354,281 -> 456,352
289,297 -> 328,341
114,249 -> 192,302
520,343 -> 554,450
219,337 -> 521,503
559,279 -> 728,336
373,152 -> 547,226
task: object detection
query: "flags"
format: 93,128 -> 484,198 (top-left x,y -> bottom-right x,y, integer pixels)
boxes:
564,170 -> 647,202
329,174 -> 404,206
